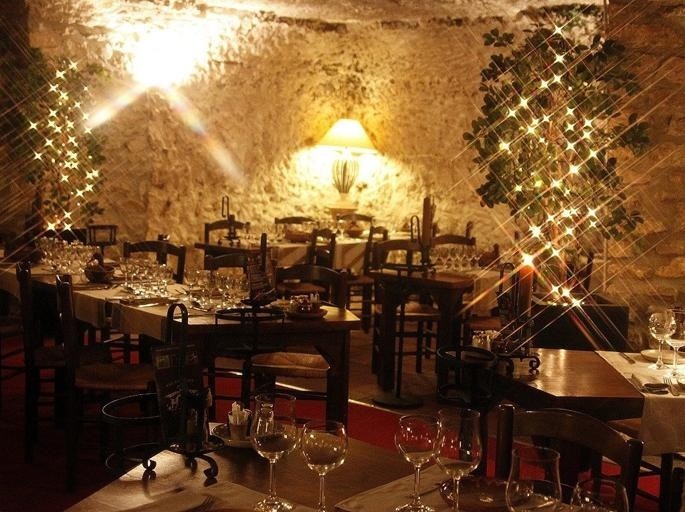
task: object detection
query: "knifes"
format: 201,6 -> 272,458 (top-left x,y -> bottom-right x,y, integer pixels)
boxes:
619,348 -> 634,365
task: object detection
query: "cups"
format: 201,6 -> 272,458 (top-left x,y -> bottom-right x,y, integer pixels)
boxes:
504,444 -> 564,512
239,222 -> 288,248
573,478 -> 628,512
228,409 -> 251,438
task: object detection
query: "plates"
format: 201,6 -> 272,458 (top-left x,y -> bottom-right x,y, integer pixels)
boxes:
640,350 -> 685,365
211,422 -> 287,449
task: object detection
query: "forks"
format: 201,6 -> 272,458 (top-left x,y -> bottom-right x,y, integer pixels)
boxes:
663,377 -> 679,399
192,495 -> 216,511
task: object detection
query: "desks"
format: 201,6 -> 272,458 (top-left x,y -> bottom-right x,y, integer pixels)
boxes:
195,229 -> 413,278
368,265 -> 517,391
442,349 -> 684,484
2,257 -> 362,435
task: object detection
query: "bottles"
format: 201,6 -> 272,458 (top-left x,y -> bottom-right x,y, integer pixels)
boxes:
471,329 -> 490,354
289,290 -> 321,313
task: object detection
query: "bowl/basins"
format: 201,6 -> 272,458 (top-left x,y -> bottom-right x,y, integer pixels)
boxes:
346,229 -> 362,238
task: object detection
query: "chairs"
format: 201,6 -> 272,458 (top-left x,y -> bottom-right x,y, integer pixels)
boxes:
0,215 -> 684,512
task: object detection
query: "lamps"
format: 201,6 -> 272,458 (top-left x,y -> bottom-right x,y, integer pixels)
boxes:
313,119 -> 384,224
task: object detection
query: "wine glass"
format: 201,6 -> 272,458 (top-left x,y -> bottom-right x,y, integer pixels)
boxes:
245,387 -> 295,512
429,247 -> 484,272
34,234 -> 92,286
393,414 -> 438,512
434,405 -> 483,512
117,255 -> 176,305
183,265 -> 249,313
297,419 -> 349,512
647,308 -> 685,377
306,217 -> 348,243
